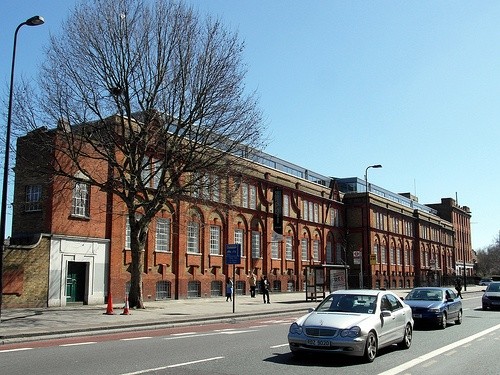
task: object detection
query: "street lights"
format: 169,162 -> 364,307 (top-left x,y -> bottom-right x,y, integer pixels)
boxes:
365,164 -> 383,289
1,13 -> 44,320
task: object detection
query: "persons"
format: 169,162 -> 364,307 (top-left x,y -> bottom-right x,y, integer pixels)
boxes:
249,274 -> 256,297
226,277 -> 234,301
455,283 -> 463,299
261,276 -> 271,304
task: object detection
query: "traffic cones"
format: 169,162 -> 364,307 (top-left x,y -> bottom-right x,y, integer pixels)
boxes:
103,291 -> 116,315
120,296 -> 132,315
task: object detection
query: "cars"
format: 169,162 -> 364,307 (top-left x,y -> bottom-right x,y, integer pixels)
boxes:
481,281 -> 500,311
478,278 -> 494,286
401,286 -> 463,330
287,288 -> 414,363
